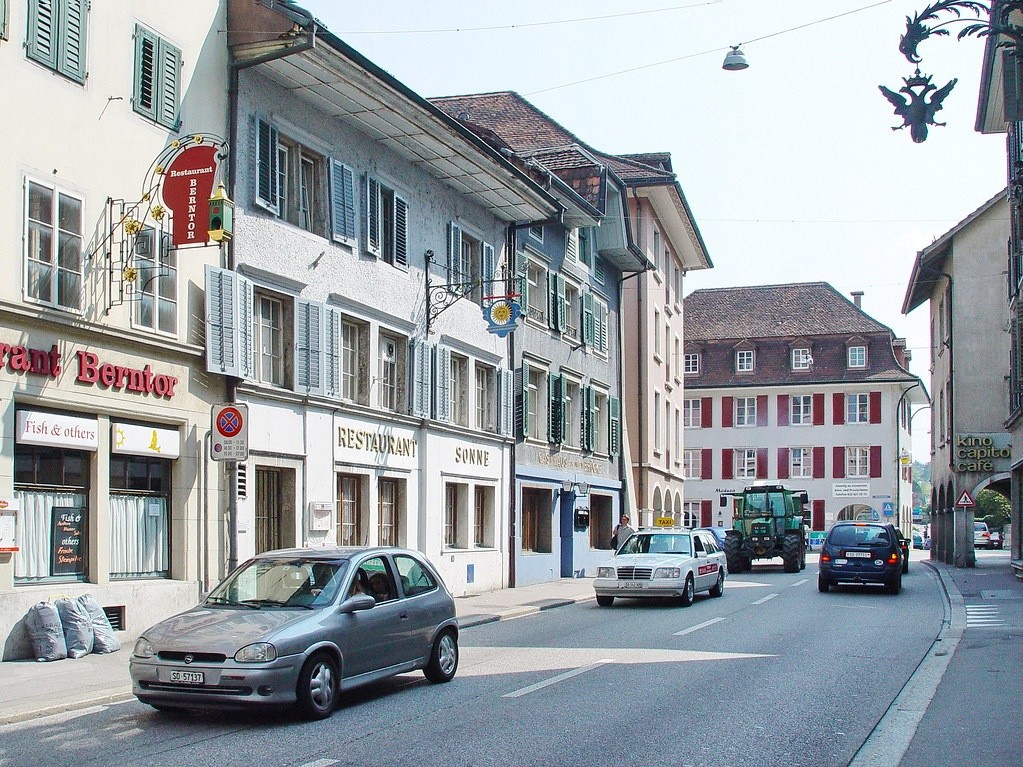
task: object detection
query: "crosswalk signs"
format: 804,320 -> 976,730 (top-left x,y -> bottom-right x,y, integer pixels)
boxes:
955,489 -> 975,507
882,501 -> 894,517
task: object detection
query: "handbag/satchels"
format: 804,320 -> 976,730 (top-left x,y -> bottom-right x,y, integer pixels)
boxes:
610,525 -> 620,550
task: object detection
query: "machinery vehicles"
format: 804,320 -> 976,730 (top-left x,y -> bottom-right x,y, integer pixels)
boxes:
721,485 -> 808,572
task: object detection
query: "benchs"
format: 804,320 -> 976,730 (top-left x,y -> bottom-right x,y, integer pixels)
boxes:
400,574 -> 431,596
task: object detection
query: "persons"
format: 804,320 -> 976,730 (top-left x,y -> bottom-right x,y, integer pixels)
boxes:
312,569 -> 415,602
613,515 -> 635,554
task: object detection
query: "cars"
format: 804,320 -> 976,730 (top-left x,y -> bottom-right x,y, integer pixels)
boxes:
693,528 -> 733,549
924,541 -> 930,550
858,528 -> 909,573
989,535 -> 1003,549
128,545 -> 459,722
591,518 -> 728,607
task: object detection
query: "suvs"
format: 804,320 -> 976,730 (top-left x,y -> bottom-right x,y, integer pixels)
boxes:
819,520 -> 904,596
913,534 -> 923,550
974,522 -> 990,549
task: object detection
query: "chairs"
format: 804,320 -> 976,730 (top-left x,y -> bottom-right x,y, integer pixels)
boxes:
656,542 -> 668,551
356,568 -> 390,601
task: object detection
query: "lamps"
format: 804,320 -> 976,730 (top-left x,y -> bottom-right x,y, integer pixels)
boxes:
723,42 -> 749,71
557,479 -> 575,498
574,481 -> 591,497
